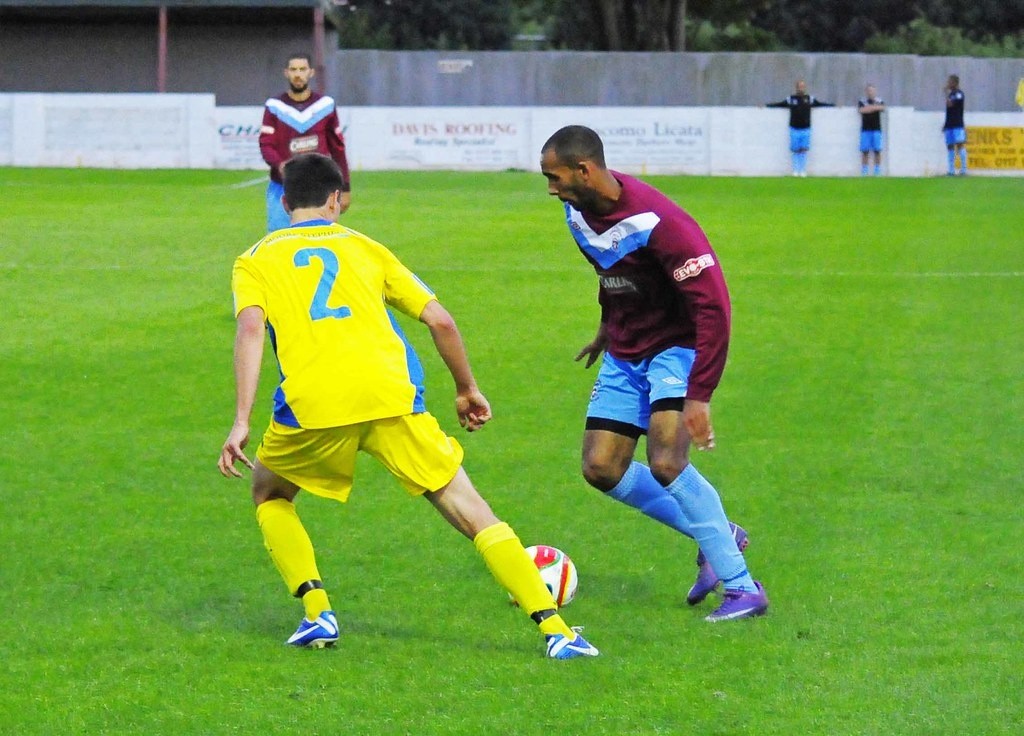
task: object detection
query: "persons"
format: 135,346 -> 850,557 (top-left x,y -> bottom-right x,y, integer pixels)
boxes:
764,80 -> 841,178
857,86 -> 885,176
217,151 -> 600,659
940,74 -> 968,176
258,52 -> 352,234
539,125 -> 770,621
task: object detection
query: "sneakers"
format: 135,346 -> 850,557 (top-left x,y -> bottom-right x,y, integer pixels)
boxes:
544,626 -> 602,660
284,611 -> 340,649
687,522 -> 749,605
703,581 -> 769,623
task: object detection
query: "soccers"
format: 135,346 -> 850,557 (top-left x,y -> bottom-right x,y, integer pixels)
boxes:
510,543 -> 582,604
